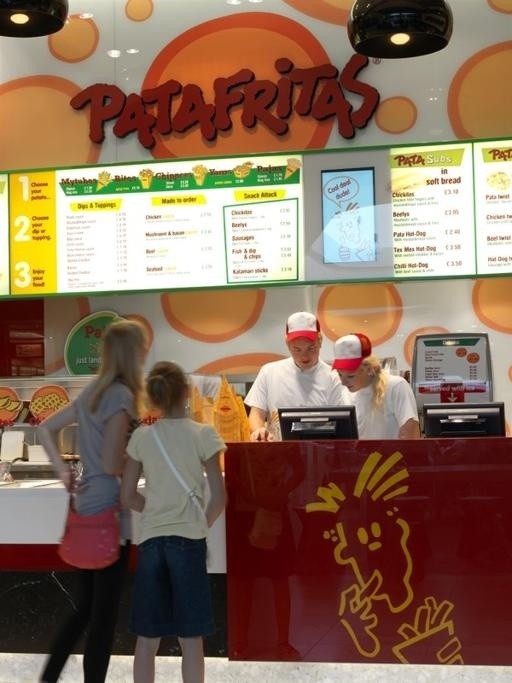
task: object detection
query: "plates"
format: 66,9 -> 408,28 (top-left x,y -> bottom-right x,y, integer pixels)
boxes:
0,482 -> 13,486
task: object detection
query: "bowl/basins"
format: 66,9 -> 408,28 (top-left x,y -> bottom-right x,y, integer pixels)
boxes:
28,446 -> 49,461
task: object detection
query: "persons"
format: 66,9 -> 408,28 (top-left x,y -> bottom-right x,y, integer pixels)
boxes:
36,319 -> 147,683
242,310 -> 347,442
333,333 -> 422,439
119,361 -> 230,683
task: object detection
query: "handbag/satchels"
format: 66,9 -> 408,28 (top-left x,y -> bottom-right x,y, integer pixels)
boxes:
58,504 -> 121,569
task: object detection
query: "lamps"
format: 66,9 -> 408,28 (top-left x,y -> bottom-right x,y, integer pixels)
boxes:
347,0 -> 453,60
0,0 -> 69,39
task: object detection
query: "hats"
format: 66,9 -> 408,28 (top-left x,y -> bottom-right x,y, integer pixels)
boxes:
286,312 -> 320,341
333,333 -> 371,369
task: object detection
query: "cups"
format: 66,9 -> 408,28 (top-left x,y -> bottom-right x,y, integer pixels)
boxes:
0,461 -> 11,480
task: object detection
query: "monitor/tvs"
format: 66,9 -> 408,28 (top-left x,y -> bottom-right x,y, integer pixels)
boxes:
423,402 -> 505,436
278,405 -> 359,439
